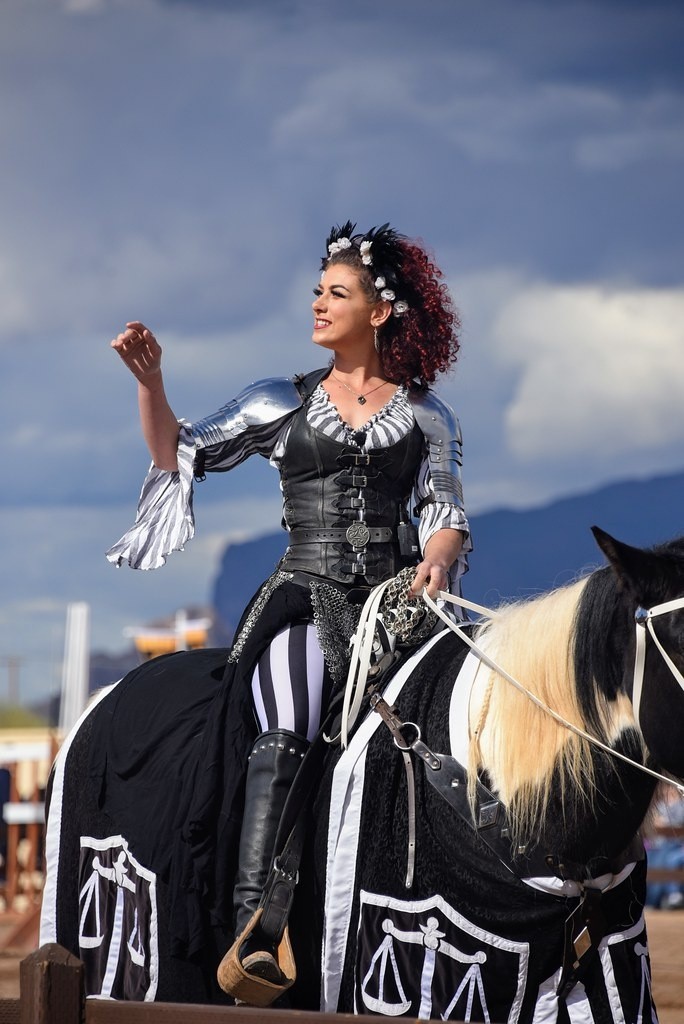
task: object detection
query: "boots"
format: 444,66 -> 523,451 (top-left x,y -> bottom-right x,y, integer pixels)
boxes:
235,729 -> 313,987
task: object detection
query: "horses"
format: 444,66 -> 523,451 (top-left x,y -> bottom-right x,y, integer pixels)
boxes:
43,527 -> 684,1024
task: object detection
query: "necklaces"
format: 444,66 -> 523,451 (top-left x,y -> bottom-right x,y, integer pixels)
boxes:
330,371 -> 390,405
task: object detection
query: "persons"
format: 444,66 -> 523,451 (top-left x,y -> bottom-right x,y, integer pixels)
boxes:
108,222 -> 473,1008
